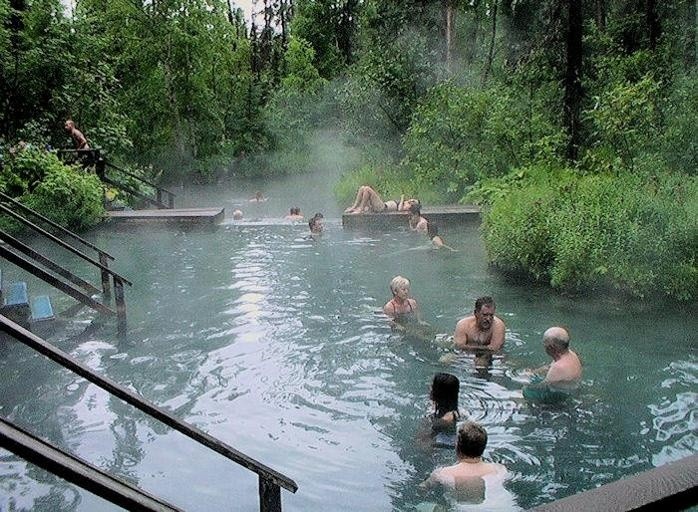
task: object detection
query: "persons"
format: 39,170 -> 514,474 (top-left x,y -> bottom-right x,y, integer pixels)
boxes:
232,210 -> 243,220
427,222 -> 458,252
438,296 -> 505,367
250,191 -> 266,202
284,207 -> 324,235
383,275 -> 430,331
415,372 -> 470,445
65,120 -> 95,168
408,205 -> 428,233
419,421 -> 509,503
343,186 -> 420,216
520,326 -> 581,404
